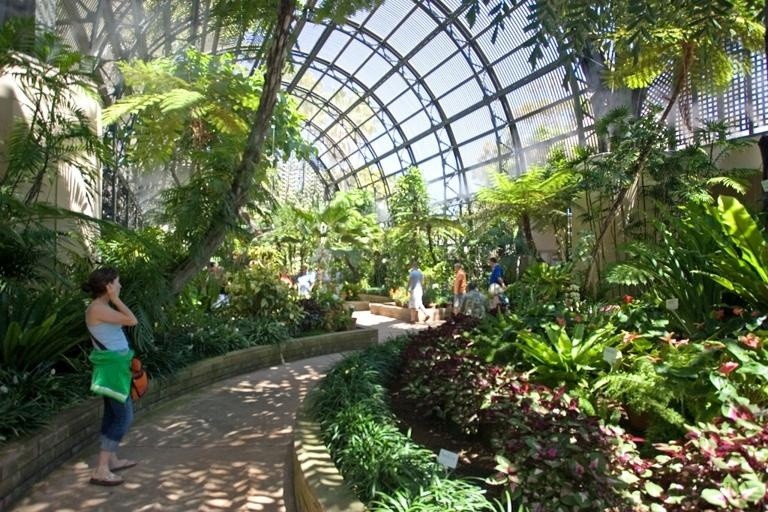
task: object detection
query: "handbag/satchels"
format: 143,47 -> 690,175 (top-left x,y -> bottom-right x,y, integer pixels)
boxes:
130,359 -> 148,401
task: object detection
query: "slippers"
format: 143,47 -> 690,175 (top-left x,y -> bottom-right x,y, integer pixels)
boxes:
109,459 -> 137,471
90,473 -> 123,485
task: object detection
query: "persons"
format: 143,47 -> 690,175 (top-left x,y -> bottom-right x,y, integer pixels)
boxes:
489,258 -> 506,289
206,260 -> 345,308
485,283 -> 509,314
406,262 -> 430,324
81,267 -> 138,485
453,264 -> 467,318
458,281 -> 488,320
